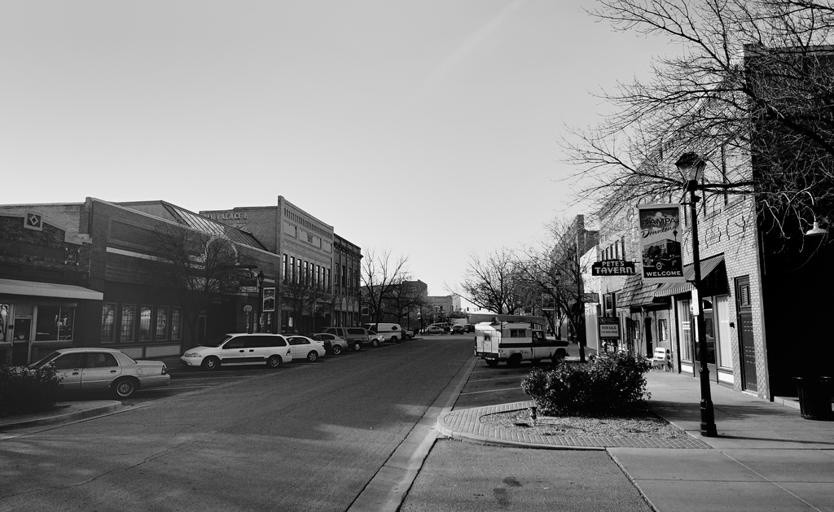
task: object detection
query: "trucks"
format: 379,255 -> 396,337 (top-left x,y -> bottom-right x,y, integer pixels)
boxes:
475,319 -> 570,369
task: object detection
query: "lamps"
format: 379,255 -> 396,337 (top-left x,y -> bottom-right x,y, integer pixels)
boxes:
804,190 -> 830,237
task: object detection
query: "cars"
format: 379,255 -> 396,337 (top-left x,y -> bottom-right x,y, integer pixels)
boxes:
419,323 -> 475,335
179,322 -> 414,371
5,347 -> 171,401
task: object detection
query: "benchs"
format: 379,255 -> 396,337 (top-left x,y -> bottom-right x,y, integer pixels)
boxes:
647,347 -> 668,371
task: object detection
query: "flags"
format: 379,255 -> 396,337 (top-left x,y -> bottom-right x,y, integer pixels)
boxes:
635,201 -> 686,283
262,287 -> 276,313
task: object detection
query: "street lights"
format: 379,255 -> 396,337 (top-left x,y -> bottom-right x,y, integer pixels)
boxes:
674,151 -> 718,437
